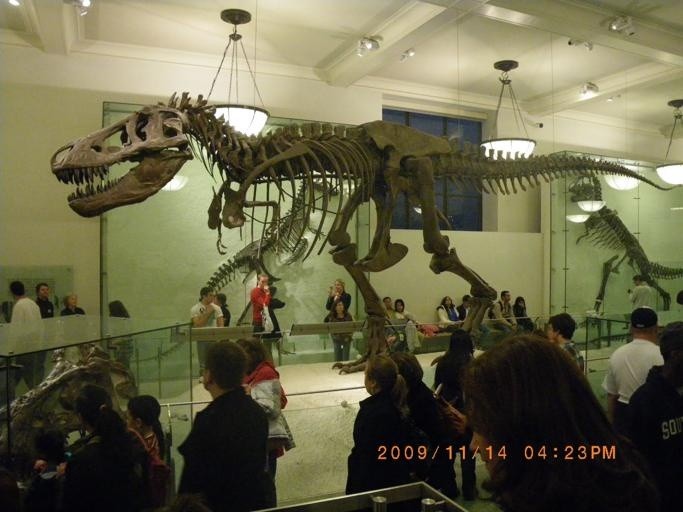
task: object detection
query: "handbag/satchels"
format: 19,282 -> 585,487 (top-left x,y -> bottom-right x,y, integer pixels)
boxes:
435,395 -> 468,435
262,305 -> 274,332
147,462 -> 166,508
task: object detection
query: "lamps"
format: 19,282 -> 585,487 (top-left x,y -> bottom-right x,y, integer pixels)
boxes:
564,161 -> 645,225
653,98 -> 683,186
479,57 -> 536,160
200,10 -> 269,138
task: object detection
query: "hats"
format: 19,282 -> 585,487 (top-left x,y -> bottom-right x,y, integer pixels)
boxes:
660,321 -> 683,353
631,308 -> 657,328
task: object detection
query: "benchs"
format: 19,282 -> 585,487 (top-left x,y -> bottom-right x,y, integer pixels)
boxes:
414,326 -> 450,352
